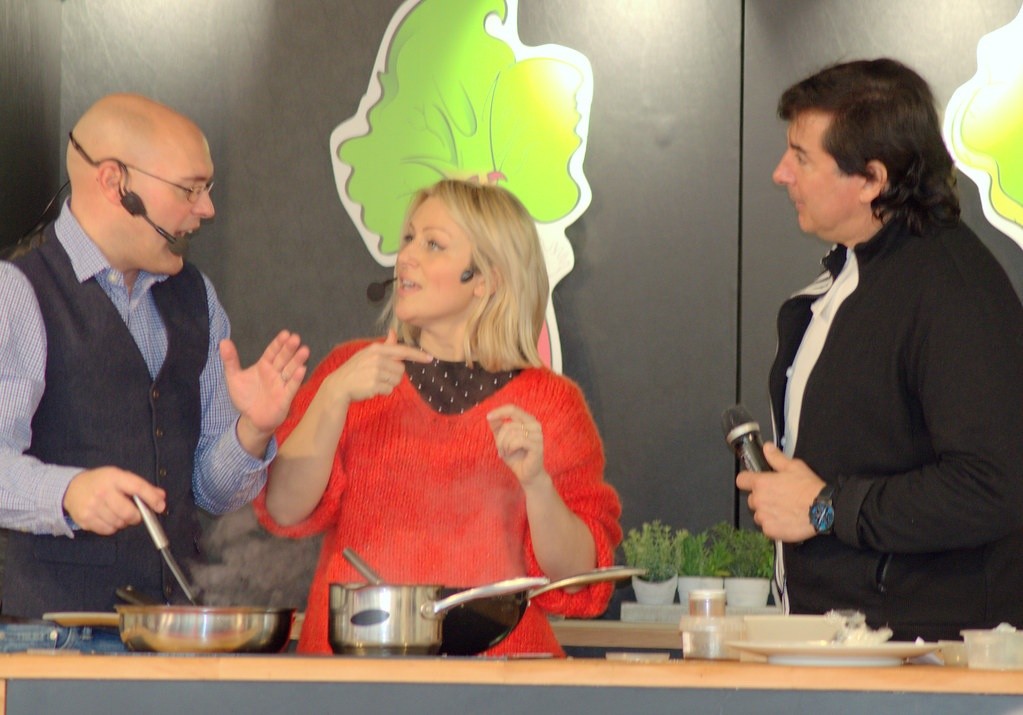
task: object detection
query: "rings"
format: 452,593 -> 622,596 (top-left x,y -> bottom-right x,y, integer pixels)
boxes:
521,422 -> 528,439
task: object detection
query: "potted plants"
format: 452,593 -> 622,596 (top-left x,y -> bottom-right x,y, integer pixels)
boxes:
619,521 -> 775,606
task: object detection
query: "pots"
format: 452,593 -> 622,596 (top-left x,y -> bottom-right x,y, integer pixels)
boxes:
327,564 -> 650,657
113,583 -> 297,653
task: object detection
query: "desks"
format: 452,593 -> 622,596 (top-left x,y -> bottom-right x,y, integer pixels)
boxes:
0,642 -> 1023,714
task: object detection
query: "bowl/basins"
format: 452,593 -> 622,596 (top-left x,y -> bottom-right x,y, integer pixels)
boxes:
961,630 -> 1023,672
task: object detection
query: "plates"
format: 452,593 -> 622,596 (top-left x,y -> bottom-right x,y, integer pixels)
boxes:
729,638 -> 945,667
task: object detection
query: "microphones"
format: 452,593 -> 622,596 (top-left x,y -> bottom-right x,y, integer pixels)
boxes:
367,278 -> 396,302
120,191 -> 191,255
720,404 -> 772,475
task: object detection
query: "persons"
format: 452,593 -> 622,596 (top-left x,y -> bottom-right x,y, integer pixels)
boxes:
0,93 -> 310,654
255,179 -> 623,659
736,57 -> 1023,642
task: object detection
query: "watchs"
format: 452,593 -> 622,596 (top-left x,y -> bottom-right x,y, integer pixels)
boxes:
808,485 -> 835,535
